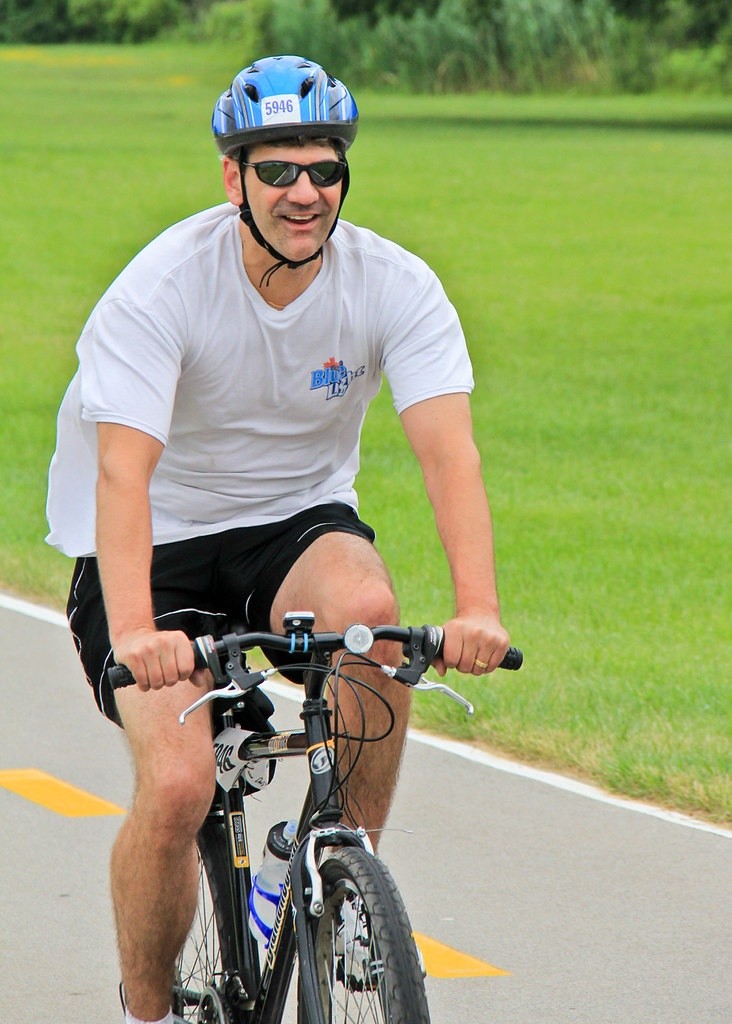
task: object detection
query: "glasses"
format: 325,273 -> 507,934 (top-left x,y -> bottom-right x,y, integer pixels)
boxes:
237,159 -> 346,187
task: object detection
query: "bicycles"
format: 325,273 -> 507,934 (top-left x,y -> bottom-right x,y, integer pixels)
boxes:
105,606 -> 525,1024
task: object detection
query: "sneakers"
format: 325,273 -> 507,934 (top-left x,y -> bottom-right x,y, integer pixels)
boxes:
332,886 -> 381,990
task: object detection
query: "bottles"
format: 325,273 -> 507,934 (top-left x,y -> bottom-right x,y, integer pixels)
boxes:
247,820 -> 298,947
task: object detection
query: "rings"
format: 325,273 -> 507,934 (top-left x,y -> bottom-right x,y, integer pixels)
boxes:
475,659 -> 489,670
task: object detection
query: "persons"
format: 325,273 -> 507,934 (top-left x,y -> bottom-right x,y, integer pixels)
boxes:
42,53 -> 509,1024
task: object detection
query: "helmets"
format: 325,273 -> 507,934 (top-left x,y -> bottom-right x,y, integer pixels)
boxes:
210,54 -> 358,154
